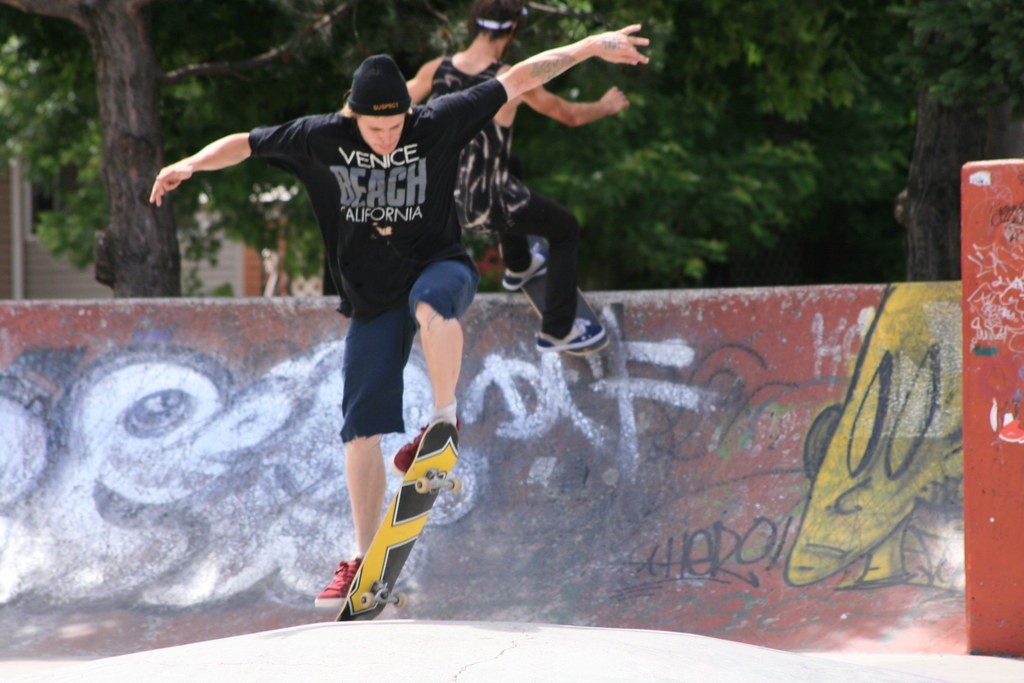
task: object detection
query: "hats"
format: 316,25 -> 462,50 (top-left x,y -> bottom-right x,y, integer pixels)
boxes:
347,54 -> 411,116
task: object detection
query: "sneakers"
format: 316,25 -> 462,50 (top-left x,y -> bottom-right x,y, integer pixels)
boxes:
314,558 -> 362,611
393,416 -> 460,477
536,319 -> 607,351
501,241 -> 554,291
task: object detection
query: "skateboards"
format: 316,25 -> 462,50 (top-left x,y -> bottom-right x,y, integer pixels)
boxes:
519,234 -> 611,356
336,416 -> 465,622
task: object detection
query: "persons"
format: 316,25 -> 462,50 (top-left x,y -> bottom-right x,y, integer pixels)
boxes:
148,25 -> 650,611
405,1 -> 630,354
